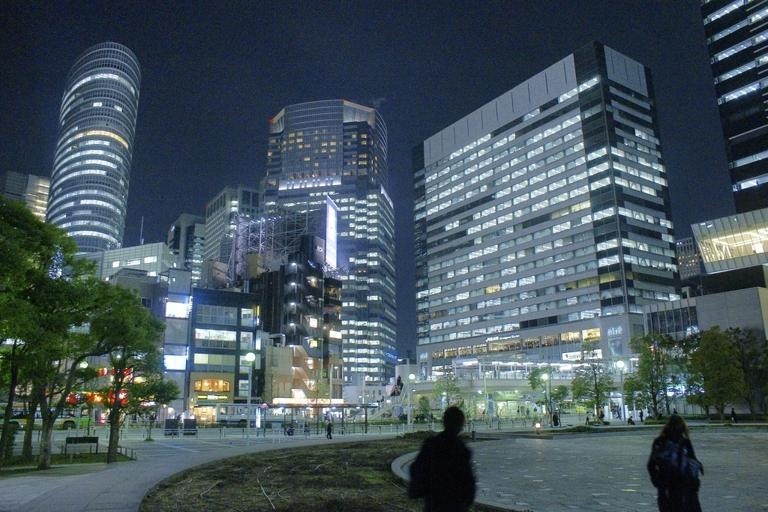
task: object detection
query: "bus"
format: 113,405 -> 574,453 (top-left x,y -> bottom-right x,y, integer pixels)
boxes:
214,402 -> 306,431
0,401 -> 98,431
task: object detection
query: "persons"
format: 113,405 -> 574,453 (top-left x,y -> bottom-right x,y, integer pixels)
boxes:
553,412 -> 559,426
639,409 -> 643,422
599,410 -> 604,422
586,412 -> 589,425
327,419 -> 332,439
732,408 -> 737,423
416,407 -> 475,512
648,415 -> 703,512
628,416 -> 635,425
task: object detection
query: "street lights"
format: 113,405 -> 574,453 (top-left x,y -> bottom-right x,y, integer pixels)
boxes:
244,351 -> 255,429
396,356 -> 410,425
356,392 -> 369,404
614,359 -> 627,421
